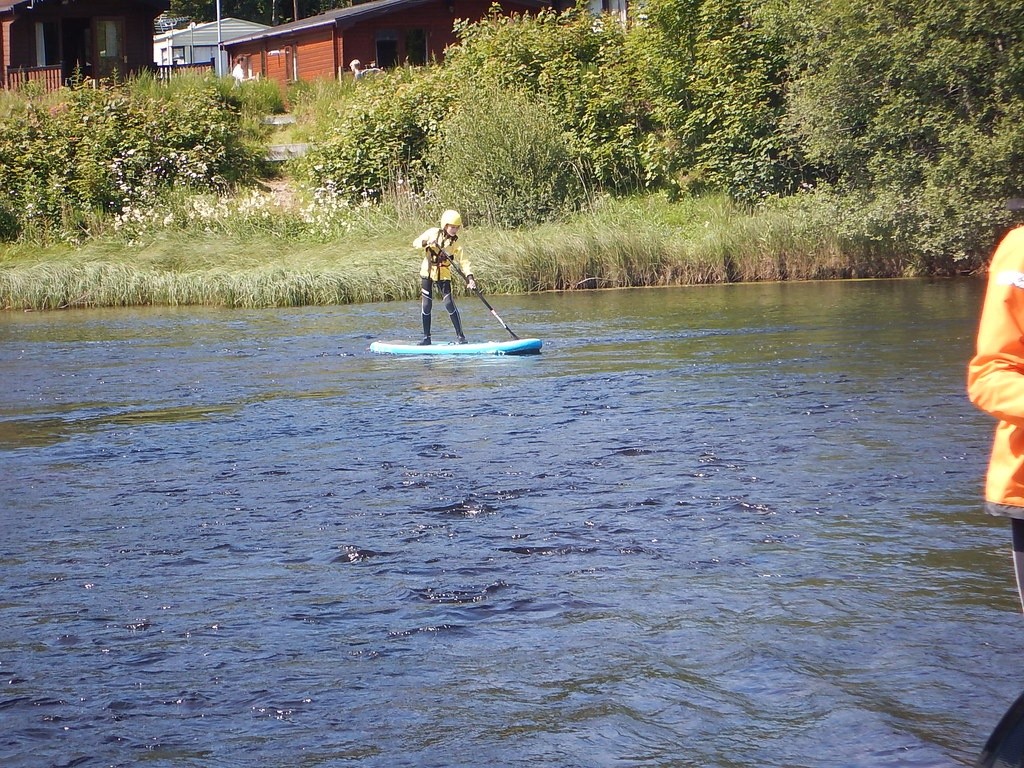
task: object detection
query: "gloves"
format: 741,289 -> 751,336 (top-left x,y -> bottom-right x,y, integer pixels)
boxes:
464,279 -> 476,290
426,238 -> 437,246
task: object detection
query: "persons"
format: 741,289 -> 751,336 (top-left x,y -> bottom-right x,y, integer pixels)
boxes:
967,226 -> 1024,689
413,209 -> 476,345
232,57 -> 244,83
349,59 -> 362,78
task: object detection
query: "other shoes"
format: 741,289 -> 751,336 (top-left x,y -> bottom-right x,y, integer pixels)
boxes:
458,336 -> 468,343
417,338 -> 430,346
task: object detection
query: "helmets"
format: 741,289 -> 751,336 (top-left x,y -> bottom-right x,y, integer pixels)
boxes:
441,210 -> 463,231
